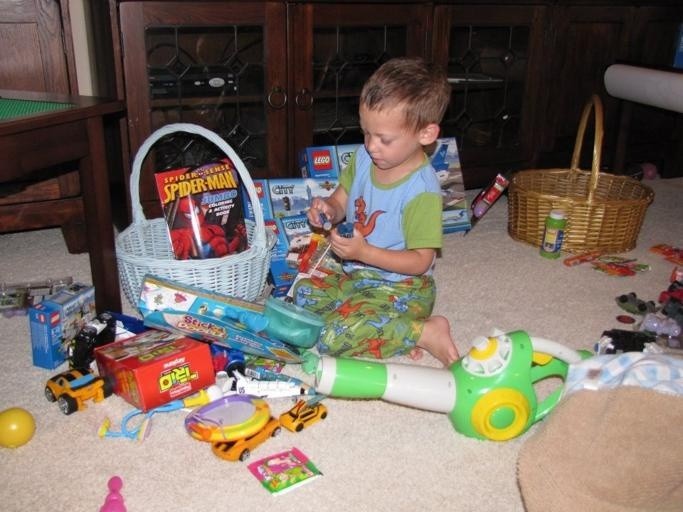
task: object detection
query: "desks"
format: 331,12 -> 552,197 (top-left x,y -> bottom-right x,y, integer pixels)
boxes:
0,89 -> 127,323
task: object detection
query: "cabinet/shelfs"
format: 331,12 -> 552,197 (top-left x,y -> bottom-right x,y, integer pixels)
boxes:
87,3 -> 681,234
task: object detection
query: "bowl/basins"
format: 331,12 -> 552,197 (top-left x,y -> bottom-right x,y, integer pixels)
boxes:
260,298 -> 325,348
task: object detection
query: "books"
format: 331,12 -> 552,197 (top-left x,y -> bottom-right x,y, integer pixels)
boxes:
245,446 -> 322,498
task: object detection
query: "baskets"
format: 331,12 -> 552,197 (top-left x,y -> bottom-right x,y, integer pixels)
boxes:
114,122 -> 277,317
506,95 -> 655,253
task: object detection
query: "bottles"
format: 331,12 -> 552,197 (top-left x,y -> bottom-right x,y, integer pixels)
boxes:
536,210 -> 566,259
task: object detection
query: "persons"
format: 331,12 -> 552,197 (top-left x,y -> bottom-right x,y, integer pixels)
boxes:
292,57 -> 461,368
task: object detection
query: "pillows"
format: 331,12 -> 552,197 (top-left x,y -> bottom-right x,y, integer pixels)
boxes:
517,330 -> 683,512
